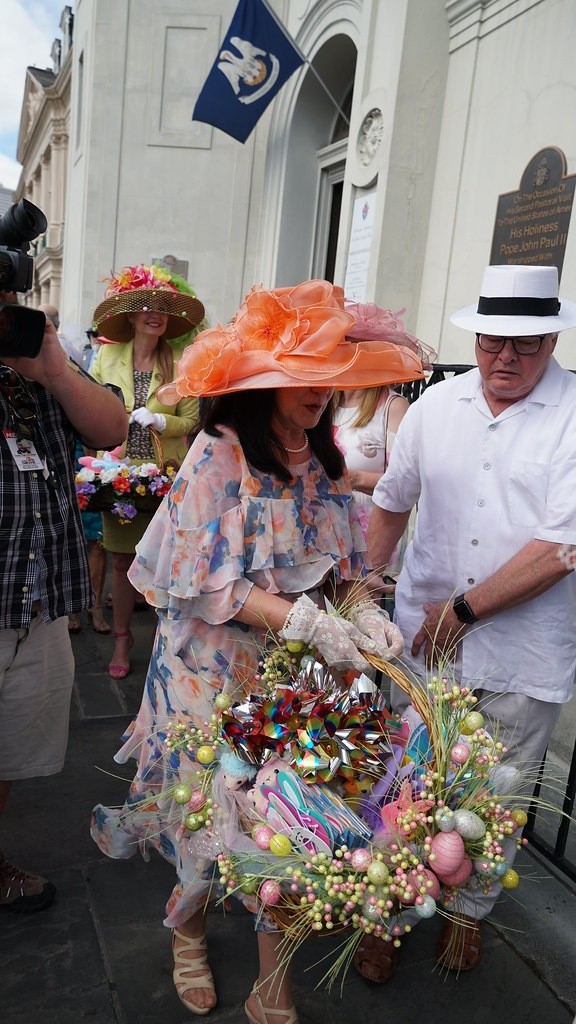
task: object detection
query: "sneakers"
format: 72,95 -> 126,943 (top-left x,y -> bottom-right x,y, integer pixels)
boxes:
0,851 -> 58,914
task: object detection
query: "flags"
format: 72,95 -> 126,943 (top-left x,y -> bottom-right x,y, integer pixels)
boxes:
190,0 -> 309,145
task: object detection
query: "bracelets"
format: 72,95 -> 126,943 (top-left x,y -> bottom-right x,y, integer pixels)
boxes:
366,568 -> 384,578
282,605 -> 296,633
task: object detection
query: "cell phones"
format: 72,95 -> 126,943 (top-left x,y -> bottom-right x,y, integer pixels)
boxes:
380,575 -> 397,622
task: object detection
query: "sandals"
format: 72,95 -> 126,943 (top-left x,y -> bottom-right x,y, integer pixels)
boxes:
171,925 -> 217,1015
353,931 -> 399,982
437,909 -> 484,970
245,979 -> 299,1024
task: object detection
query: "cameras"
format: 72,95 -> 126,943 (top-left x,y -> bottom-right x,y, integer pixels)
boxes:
0,197 -> 48,359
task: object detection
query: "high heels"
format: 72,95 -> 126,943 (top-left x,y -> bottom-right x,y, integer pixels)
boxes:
87,606 -> 111,634
68,614 -> 82,634
108,628 -> 134,679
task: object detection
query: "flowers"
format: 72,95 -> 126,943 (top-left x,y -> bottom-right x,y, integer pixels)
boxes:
73,463 -> 178,527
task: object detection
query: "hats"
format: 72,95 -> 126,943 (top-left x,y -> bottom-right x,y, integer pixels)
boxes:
86,324 -> 98,332
93,264 -> 206,343
340,297 -> 437,372
155,278 -> 428,408
451,264 -> 576,337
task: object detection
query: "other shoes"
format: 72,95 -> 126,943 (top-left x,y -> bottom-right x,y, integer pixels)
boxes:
104,589 -> 150,611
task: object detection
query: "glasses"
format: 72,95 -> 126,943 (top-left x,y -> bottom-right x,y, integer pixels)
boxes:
90,332 -> 98,338
475,333 -> 553,356
0,364 -> 38,420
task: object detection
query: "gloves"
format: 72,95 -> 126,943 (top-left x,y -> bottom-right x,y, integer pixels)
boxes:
129,406 -> 166,431
350,601 -> 405,666
276,593 -> 378,678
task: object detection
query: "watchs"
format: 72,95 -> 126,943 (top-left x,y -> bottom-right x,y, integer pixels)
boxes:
452,592 -> 479,625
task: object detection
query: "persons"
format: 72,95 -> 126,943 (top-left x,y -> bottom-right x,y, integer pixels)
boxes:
331,302 -> 438,606
350,267 -> 575,985
37,303 -> 84,376
68,441 -> 111,634
80,324 -> 104,373
0,285 -> 129,915
82,258 -> 203,679
90,280 -> 428,1024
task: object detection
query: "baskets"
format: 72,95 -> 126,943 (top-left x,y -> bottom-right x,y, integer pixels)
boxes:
79,419 -> 165,513
208,644 -> 453,940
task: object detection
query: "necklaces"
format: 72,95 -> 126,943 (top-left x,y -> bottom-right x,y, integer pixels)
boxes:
270,430 -> 308,453
